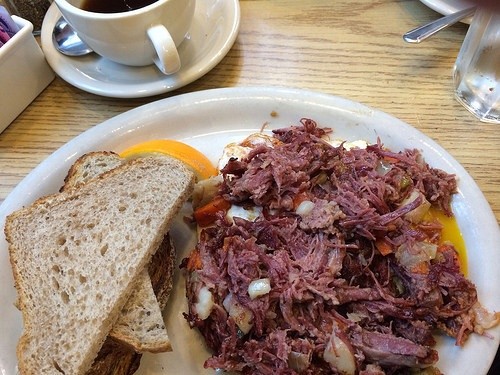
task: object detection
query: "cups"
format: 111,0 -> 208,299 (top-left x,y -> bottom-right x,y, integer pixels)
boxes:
52,0 -> 200,75
452,0 -> 500,125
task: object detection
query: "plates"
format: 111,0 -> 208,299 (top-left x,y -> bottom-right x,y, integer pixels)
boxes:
40,0 -> 242,99
419,0 -> 479,26
0,87 -> 500,375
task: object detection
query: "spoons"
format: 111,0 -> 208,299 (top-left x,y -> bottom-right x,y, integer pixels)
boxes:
52,15 -> 94,57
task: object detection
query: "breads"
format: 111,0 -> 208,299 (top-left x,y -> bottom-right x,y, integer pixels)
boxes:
5,149 -> 198,374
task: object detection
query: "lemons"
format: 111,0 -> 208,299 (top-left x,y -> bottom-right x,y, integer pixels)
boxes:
117,140 -> 218,185
